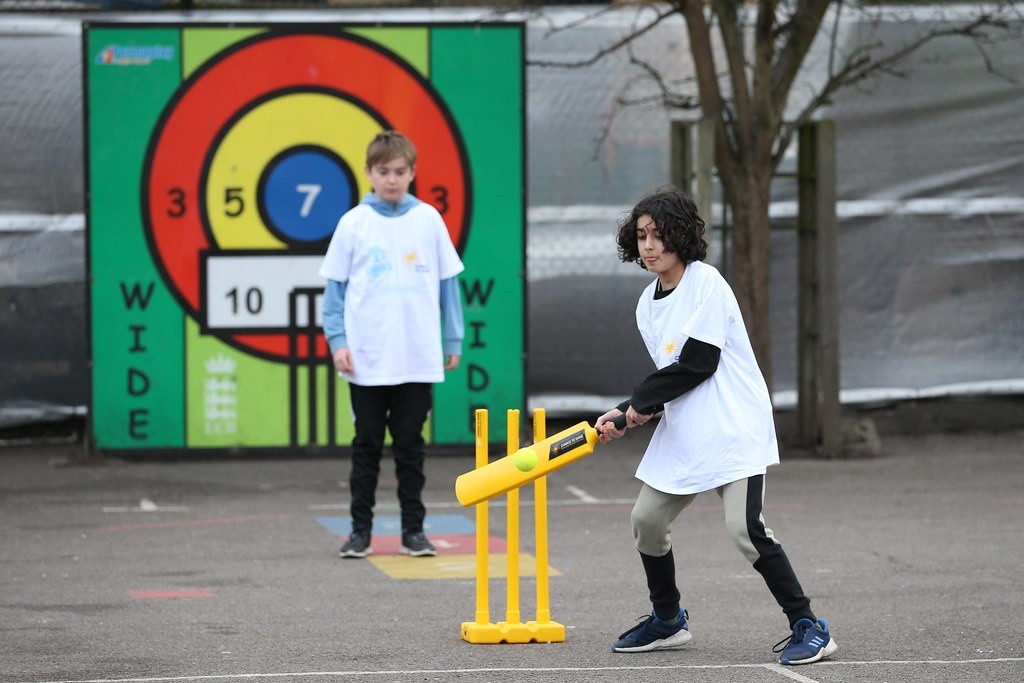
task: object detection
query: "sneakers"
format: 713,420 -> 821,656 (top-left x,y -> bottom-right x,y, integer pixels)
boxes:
339,532 -> 373,557
400,532 -> 436,556
611,608 -> 692,652
772,618 -> 838,664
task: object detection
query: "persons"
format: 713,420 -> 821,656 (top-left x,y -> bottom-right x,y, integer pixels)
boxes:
594,192 -> 839,664
319,131 -> 464,557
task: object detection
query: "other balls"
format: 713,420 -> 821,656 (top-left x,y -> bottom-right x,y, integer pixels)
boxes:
514,446 -> 538,471
